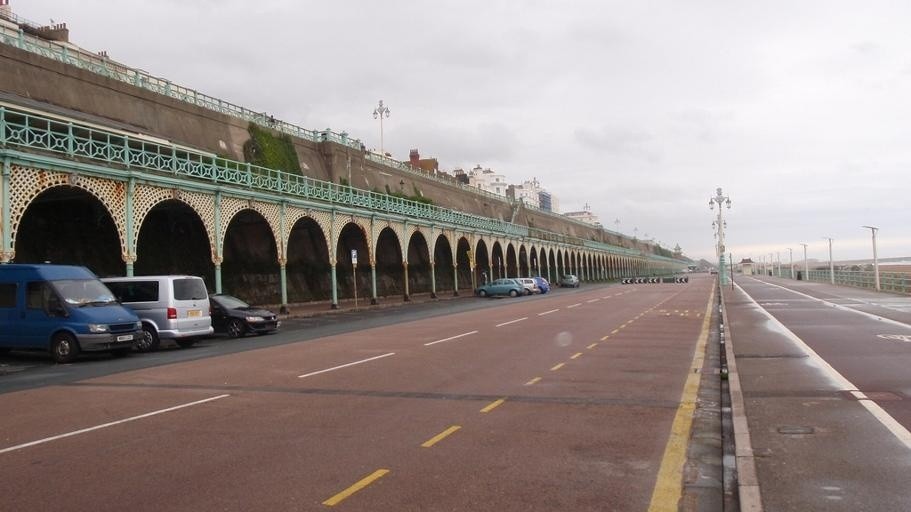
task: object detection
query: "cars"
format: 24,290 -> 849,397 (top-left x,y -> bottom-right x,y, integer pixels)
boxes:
561,275 -> 579,288
474,277 -> 551,298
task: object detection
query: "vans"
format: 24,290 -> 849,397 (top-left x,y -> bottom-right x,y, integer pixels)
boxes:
0,263 -> 282,364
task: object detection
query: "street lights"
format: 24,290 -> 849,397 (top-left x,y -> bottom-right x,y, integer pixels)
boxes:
709,187 -> 731,285
801,242 -> 810,280
758,251 -> 781,276
822,235 -> 834,283
786,247 -> 794,278
862,224 -> 880,291
373,99 -> 390,155
